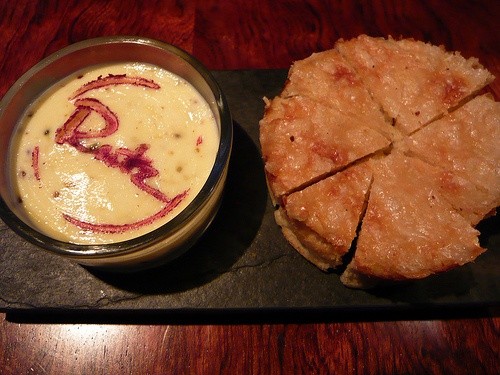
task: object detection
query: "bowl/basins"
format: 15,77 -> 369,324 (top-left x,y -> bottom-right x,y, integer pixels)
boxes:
1,37 -> 233,273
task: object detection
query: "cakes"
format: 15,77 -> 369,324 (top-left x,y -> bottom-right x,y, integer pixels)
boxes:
258,35 -> 500,284
